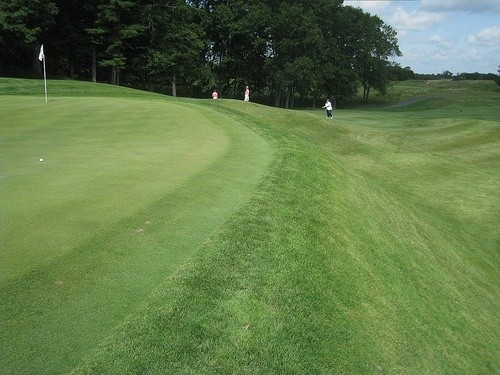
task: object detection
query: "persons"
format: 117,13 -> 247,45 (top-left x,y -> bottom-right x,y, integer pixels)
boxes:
322,99 -> 333,119
212,90 -> 218,100
244,86 -> 249,102
